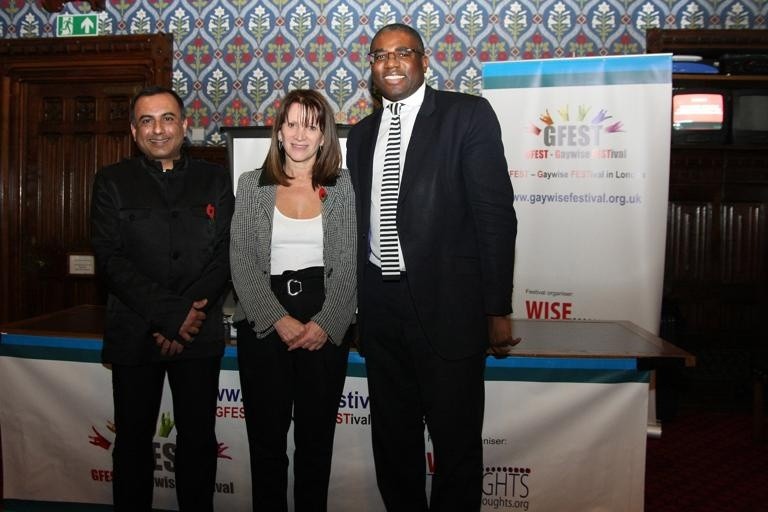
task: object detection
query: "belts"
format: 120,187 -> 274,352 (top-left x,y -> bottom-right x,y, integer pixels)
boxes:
269,274 -> 326,299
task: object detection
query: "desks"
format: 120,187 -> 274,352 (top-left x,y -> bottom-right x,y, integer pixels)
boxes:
1,304 -> 694,512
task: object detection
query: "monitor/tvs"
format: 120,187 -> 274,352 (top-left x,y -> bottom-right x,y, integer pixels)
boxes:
671,86 -> 730,144
731,88 -> 768,144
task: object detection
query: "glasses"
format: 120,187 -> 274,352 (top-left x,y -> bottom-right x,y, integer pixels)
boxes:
366,47 -> 425,62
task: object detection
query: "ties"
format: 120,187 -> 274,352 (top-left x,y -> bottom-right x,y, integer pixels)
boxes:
379,101 -> 405,282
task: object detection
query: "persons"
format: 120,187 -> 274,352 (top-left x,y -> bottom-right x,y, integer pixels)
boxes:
346,22 -> 524,512
83,84 -> 233,511
225,88 -> 360,512
